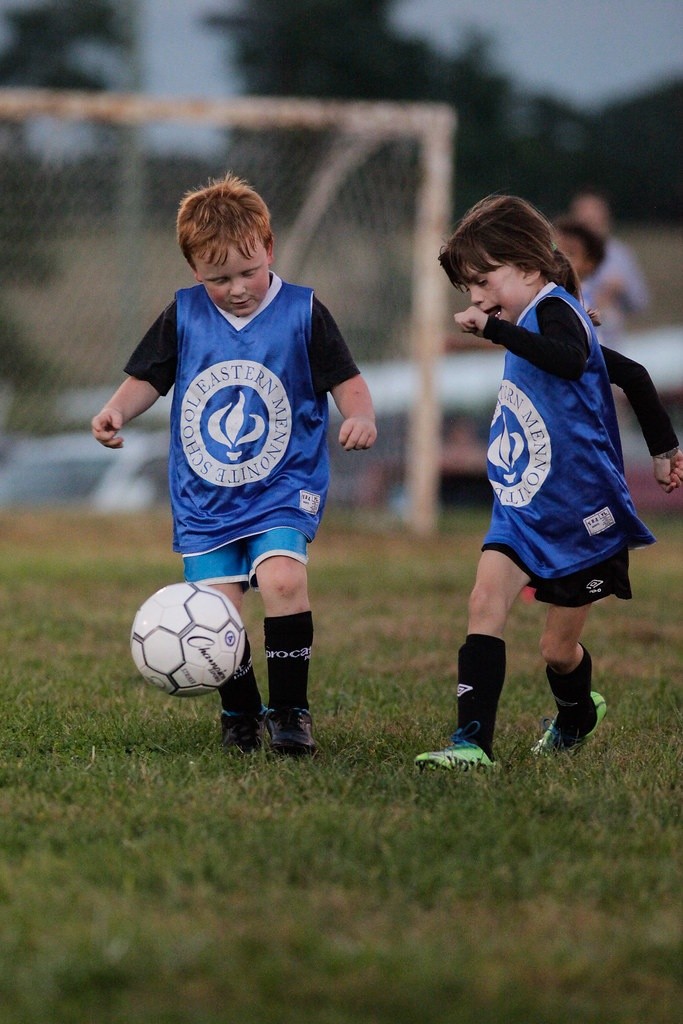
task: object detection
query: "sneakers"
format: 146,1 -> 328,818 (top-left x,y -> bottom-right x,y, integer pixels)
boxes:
264,708 -> 316,756
221,704 -> 266,756
414,720 -> 503,774
530,691 -> 607,757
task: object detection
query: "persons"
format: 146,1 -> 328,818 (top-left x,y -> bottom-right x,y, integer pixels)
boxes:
544,188 -> 654,352
418,193 -> 683,769
90,177 -> 380,758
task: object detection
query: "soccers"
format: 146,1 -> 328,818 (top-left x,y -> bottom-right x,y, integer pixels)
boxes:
128,580 -> 247,699
